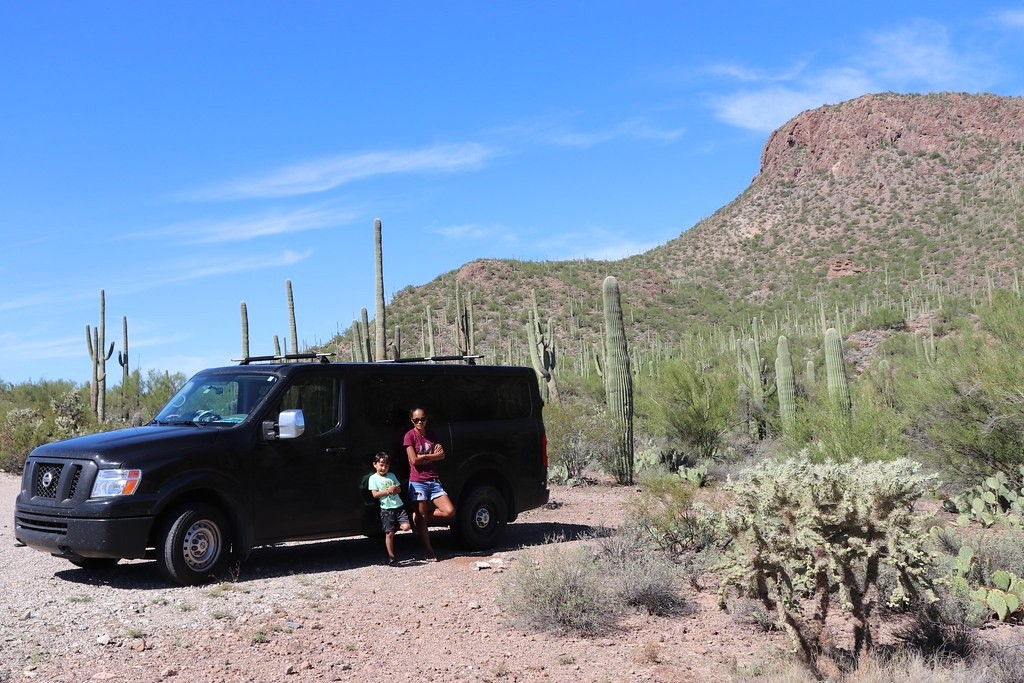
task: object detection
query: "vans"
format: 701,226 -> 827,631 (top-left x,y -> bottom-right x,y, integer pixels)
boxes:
13,352 -> 549,586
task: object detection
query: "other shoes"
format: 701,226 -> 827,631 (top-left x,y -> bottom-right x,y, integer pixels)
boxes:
424,553 -> 439,562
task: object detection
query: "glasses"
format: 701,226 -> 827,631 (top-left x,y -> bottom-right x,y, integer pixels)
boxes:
412,416 -> 428,424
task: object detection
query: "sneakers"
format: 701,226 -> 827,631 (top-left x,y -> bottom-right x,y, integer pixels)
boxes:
387,555 -> 401,567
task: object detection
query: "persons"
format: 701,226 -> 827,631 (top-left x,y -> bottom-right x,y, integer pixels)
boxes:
368,451 -> 411,566
402,405 -> 456,562
